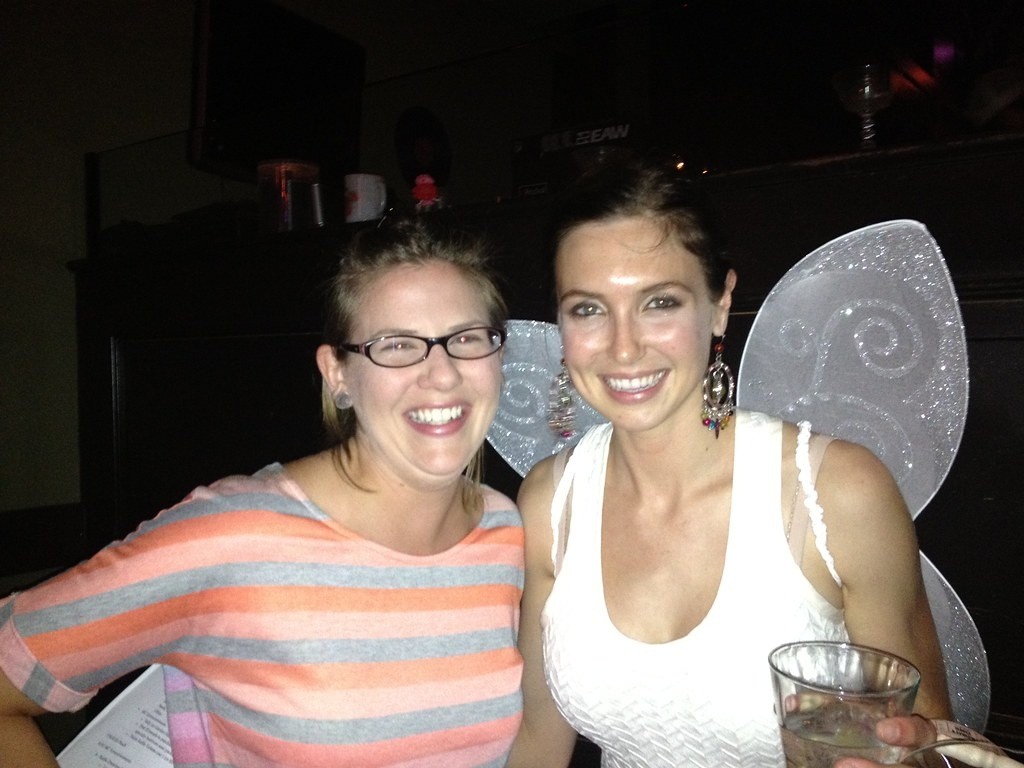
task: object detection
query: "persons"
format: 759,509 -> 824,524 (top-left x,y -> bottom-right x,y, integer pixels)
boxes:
500,147 -> 1024,768
0,209 -> 525,767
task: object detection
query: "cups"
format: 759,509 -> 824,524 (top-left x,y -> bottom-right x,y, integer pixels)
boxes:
346,173 -> 385,223
770,639 -> 920,768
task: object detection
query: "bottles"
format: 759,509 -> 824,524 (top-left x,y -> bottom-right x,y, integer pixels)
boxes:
303,180 -> 332,230
850,57 -> 894,148
